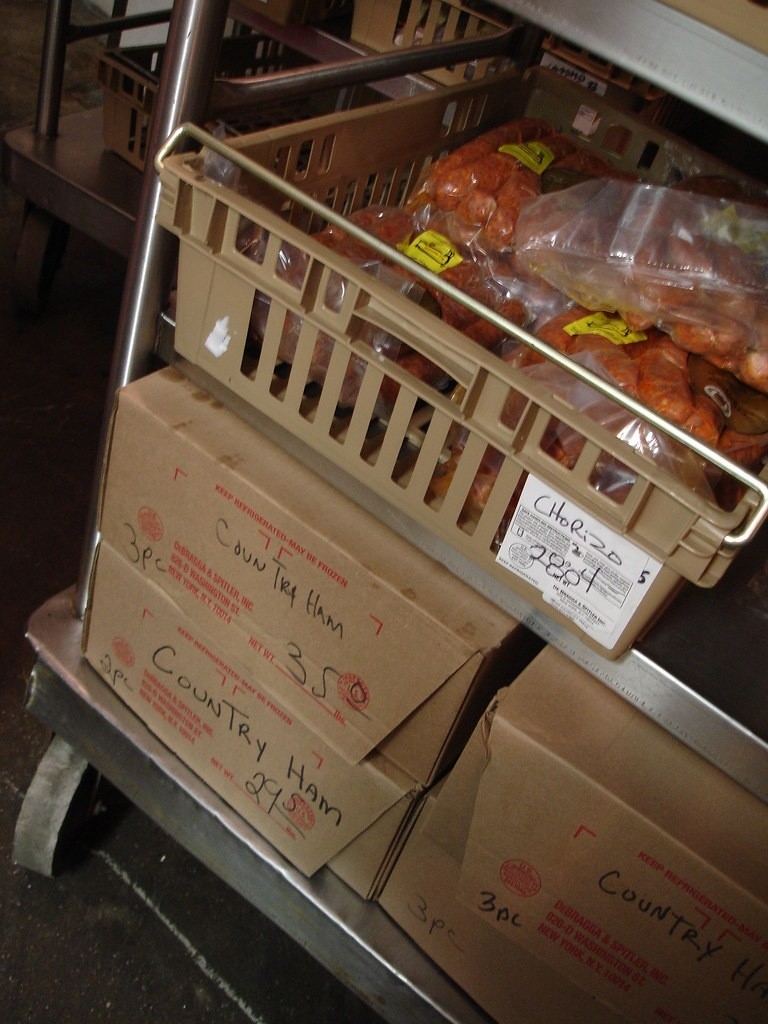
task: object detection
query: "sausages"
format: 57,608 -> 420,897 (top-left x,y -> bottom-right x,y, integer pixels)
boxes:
249,111 -> 768,547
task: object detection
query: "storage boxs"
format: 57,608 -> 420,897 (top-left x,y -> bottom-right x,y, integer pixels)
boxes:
82,0 -> 768,1024
